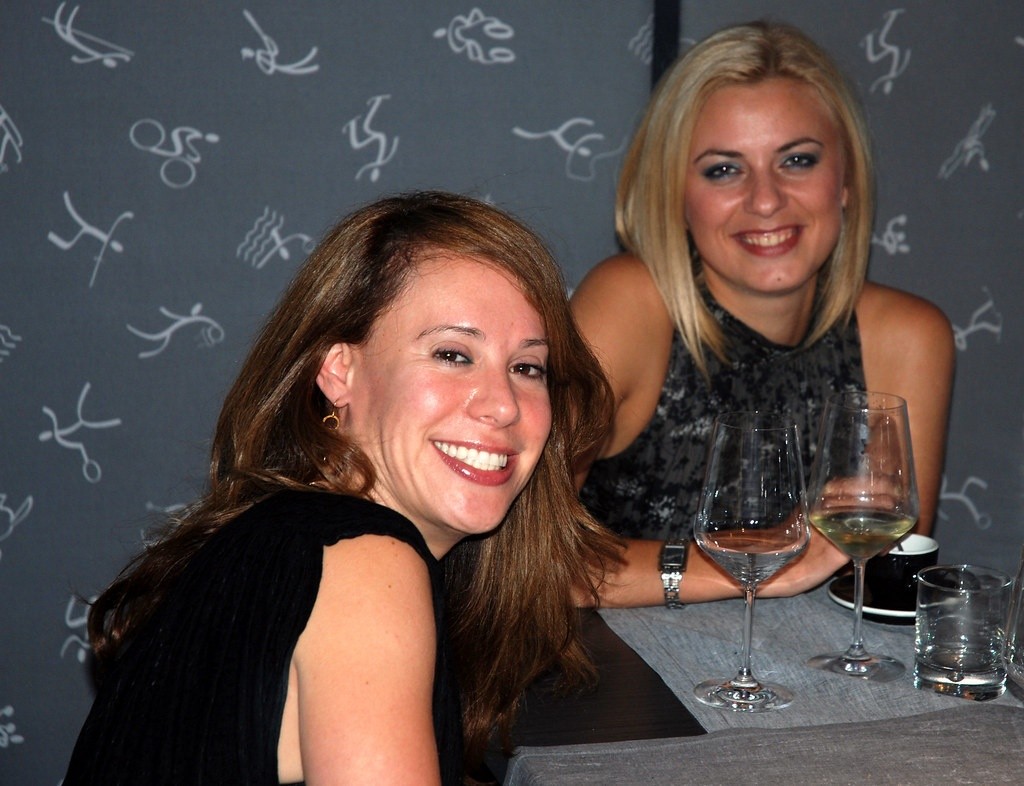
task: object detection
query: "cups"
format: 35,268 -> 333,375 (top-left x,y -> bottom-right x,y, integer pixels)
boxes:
913,564 -> 1012,702
867,532 -> 940,607
1003,549 -> 1024,690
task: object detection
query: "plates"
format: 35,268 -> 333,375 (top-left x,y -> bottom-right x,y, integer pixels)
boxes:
825,569 -> 978,618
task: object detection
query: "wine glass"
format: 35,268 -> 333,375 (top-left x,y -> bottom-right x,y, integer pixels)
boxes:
809,392 -> 918,687
691,409 -> 813,712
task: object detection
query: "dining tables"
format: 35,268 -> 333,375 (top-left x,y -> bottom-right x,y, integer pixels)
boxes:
477,555 -> 1023,786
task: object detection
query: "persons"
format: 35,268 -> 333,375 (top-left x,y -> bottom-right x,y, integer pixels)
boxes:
61,188 -> 619,786
521,14 -> 960,619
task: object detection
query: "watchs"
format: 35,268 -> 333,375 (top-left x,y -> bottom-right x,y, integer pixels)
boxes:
655,530 -> 690,613
